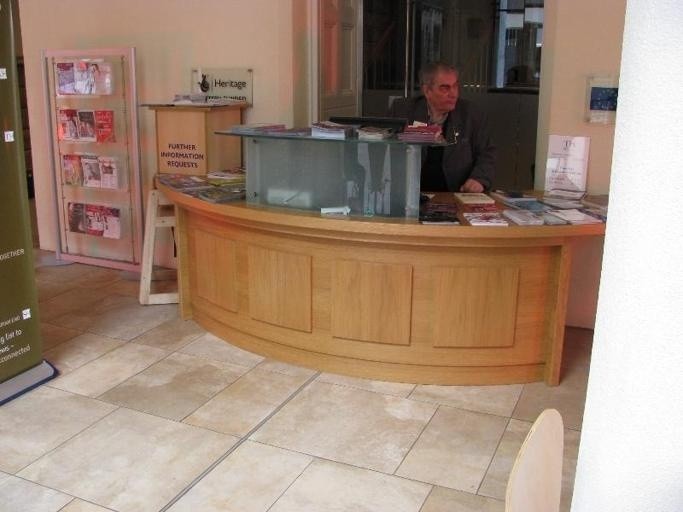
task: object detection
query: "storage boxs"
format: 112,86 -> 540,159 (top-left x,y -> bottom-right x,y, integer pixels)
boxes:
149,99 -> 243,174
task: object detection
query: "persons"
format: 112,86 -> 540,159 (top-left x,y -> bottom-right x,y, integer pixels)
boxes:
388,59 -> 506,192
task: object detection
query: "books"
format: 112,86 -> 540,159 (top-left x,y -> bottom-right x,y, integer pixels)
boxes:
158,167 -> 245,204
419,188 -> 609,227
232,121 -> 443,143
53,58 -> 123,240
